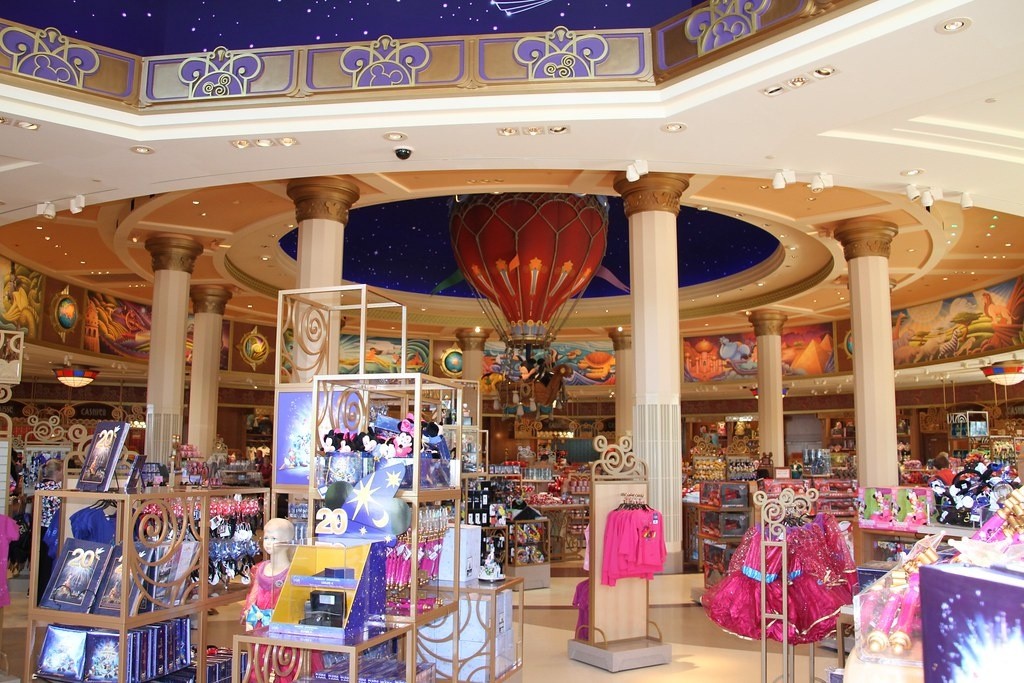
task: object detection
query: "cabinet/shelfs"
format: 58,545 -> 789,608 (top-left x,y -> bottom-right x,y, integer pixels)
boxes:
503,518 -> 551,592
824,409 -> 921,460
307,373 -> 463,683
696,480 -> 759,545
566,491 -> 590,549
24,484 -> 270,683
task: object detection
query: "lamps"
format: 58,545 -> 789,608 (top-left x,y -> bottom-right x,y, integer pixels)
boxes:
772,170 -> 834,193
749,387 -> 790,400
960,192 -> 974,209
32,201 -> 56,219
979,364 -> 1024,385
905,184 -> 944,206
51,364 -> 101,388
624,159 -> 649,182
68,194 -> 86,214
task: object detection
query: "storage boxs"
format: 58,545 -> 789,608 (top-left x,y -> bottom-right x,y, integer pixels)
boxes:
857,485 -> 935,533
416,589 -> 514,683
438,523 -> 482,582
700,478 -> 860,590
268,536 -> 389,646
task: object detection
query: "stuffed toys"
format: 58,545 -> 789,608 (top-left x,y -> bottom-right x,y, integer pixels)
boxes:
319,412 -> 443,457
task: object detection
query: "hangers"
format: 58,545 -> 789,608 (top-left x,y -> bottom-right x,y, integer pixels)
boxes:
89,487 -> 117,518
615,492 -> 654,513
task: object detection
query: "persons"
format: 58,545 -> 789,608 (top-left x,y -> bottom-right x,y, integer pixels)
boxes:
256,450 -> 264,463
36,459 -> 63,595
927,455 -> 957,489
260,454 -> 272,488
241,518 -> 324,683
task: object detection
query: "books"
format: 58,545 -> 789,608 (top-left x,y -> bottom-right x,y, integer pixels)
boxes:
37,538 -> 248,683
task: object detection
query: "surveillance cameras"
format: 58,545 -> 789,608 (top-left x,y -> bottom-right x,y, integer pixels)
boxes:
392,146 -> 415,160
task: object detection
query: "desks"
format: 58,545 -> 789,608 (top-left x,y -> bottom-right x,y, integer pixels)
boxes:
420,576 -> 525,683
